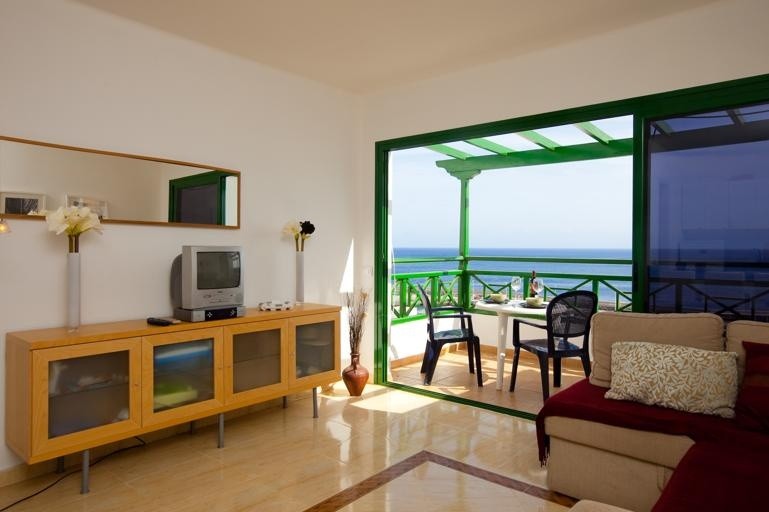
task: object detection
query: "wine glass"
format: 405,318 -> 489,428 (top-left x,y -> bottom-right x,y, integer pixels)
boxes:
511,275 -> 522,305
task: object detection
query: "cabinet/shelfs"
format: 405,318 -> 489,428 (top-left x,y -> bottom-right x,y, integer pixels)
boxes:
5,299 -> 343,495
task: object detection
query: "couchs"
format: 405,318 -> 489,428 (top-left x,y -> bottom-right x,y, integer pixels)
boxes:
538,308 -> 767,512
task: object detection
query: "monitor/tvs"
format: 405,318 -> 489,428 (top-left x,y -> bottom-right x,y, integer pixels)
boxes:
170,244 -> 245,310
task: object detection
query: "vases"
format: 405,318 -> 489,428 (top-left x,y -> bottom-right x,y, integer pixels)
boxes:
63,250 -> 85,334
295,247 -> 305,308
342,354 -> 371,399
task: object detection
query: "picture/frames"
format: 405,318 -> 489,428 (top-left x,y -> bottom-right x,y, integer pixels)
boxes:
63,192 -> 112,220
1,191 -> 48,215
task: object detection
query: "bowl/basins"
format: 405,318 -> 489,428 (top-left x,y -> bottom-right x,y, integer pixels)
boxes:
525,297 -> 545,307
490,294 -> 506,303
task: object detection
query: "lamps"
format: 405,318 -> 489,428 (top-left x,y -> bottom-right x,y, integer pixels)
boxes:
0,218 -> 11,234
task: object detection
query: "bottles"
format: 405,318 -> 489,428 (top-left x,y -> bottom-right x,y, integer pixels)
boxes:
529,270 -> 539,297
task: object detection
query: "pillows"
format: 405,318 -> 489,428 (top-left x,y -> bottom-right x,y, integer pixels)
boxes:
602,338 -> 742,422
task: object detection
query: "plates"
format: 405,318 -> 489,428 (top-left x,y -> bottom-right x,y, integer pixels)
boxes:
520,302 -> 548,309
485,299 -> 512,304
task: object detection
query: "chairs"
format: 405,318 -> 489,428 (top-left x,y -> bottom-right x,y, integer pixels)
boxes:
410,278 -> 487,388
509,288 -> 596,405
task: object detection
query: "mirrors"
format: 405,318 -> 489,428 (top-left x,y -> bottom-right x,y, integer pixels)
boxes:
1,134 -> 243,232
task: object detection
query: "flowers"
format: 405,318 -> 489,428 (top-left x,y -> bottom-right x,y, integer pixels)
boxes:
43,202 -> 104,253
283,220 -> 316,251
344,288 -> 371,354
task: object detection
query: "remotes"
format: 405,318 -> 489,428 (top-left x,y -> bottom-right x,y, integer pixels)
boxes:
159,316 -> 182,324
147,317 -> 169,326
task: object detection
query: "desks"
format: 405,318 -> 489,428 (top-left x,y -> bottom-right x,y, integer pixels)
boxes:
474,297 -> 568,394
